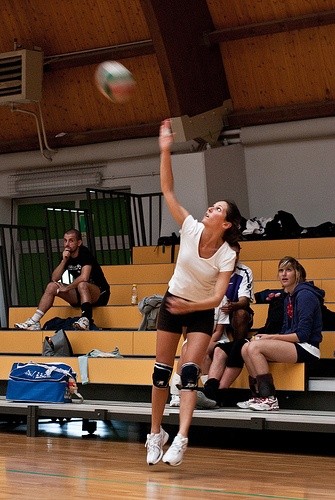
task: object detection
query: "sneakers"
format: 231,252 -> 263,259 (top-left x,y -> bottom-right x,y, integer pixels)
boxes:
162,434 -> 188,466
195,391 -> 219,409
72,317 -> 89,331
236,397 -> 279,410
145,425 -> 169,465
14,318 -> 41,330
169,395 -> 180,407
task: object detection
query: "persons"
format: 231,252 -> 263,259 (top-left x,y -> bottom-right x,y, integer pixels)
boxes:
241,256 -> 326,414
171,238 -> 257,407
14,228 -> 111,332
146,119 -> 245,467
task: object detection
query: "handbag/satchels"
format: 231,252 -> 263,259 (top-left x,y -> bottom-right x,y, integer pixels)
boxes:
158,232 -> 178,245
6,361 -> 83,404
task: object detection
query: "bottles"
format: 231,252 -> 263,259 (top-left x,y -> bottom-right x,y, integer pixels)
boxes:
131,284 -> 138,305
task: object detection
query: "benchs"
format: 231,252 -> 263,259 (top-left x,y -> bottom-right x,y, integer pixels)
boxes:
1,236 -> 335,394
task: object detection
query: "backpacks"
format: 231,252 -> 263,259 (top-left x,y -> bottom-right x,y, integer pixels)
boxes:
42,328 -> 72,356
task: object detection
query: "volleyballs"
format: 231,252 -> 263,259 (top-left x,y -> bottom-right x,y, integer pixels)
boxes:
95,60 -> 137,103
